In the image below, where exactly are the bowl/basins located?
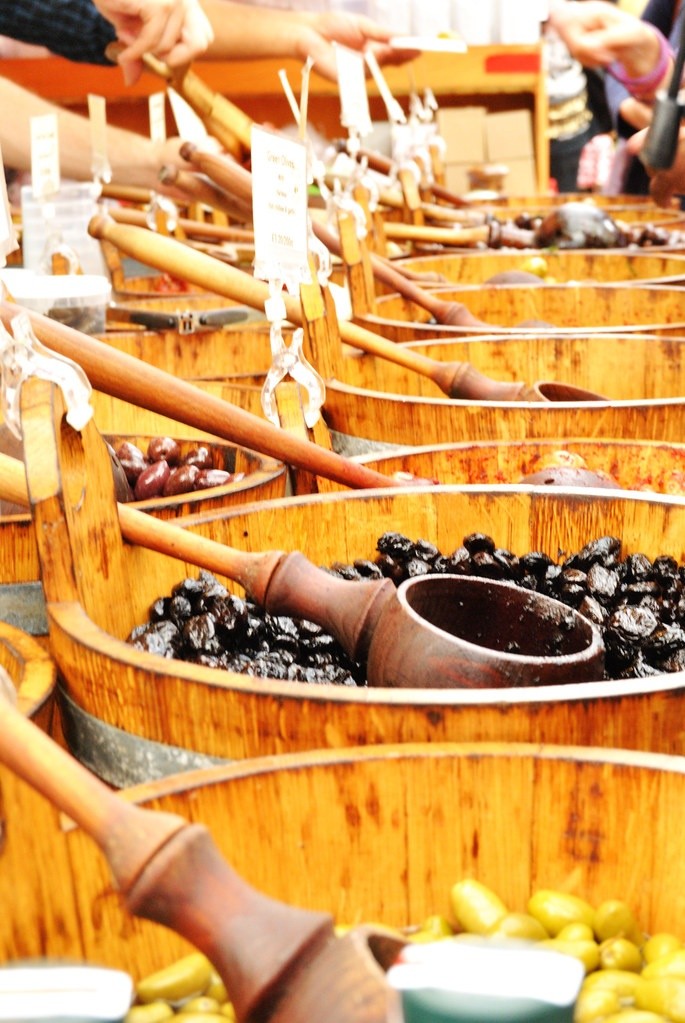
[3,178,683,1023]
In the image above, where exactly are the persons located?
[0,1,685,270]
